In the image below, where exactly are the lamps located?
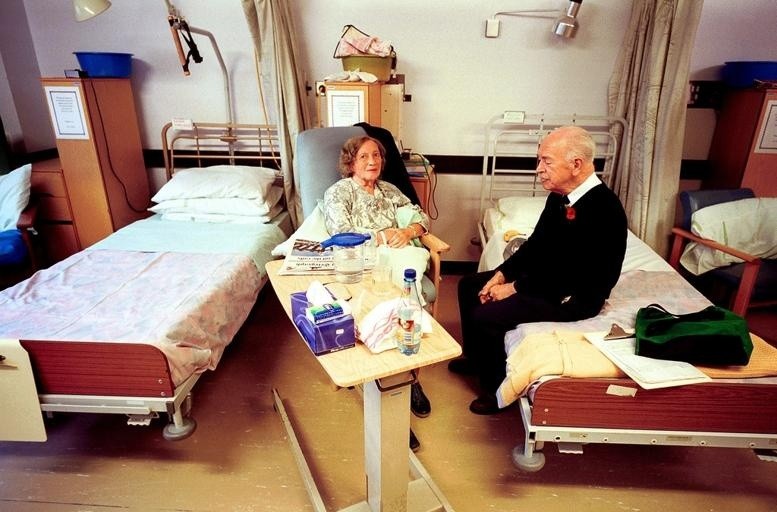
[482,1,580,47]
[70,0,202,74]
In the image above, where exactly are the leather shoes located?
[409,426,421,454]
[469,393,503,414]
[447,358,472,374]
[410,380,431,419]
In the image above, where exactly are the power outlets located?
[314,81,326,96]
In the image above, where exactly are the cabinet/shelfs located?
[318,78,381,130]
[399,162,437,214]
[26,156,79,264]
[38,75,153,271]
[706,86,776,197]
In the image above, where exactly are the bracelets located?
[408,225,417,238]
[379,231,387,245]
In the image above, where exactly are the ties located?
[559,194,570,209]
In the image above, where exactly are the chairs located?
[290,123,449,325]
[667,184,775,322]
[0,115,40,289]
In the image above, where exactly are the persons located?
[448,126,627,415]
[324,136,430,453]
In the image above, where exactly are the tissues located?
[290,280,355,356]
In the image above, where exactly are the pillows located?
[159,205,284,226]
[677,195,775,277]
[0,162,33,234]
[493,196,548,228]
[149,162,279,204]
[145,186,286,217]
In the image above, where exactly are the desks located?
[265,253,466,512]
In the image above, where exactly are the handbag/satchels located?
[633,298,754,369]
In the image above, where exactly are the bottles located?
[396,269,423,356]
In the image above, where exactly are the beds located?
[0,119,296,447]
[476,110,775,475]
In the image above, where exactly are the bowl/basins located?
[71,51,135,79]
